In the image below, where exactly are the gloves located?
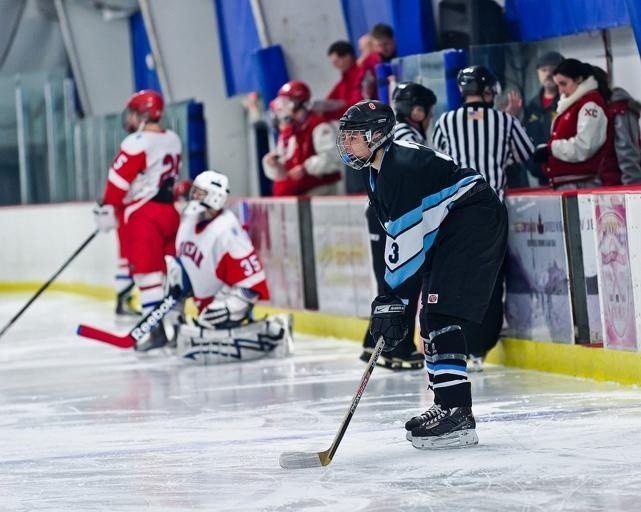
[369,296,409,353]
[195,298,231,329]
[93,201,119,232]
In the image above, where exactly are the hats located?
[535,51,566,69]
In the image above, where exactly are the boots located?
[116,294,142,317]
[132,324,178,350]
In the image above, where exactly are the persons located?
[335,100,508,449]
[609,87,641,186]
[524,52,563,139]
[165,170,294,363]
[357,24,397,71]
[535,59,623,189]
[262,82,345,196]
[320,41,375,195]
[432,65,550,373]
[92,88,188,356]
[359,82,439,369]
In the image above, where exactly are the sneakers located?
[405,404,448,432]
[268,313,292,355]
[364,347,425,363]
[411,405,477,436]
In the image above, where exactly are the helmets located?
[391,82,437,106]
[335,99,395,169]
[278,80,309,102]
[126,89,164,122]
[455,63,497,92]
[190,169,230,211]
